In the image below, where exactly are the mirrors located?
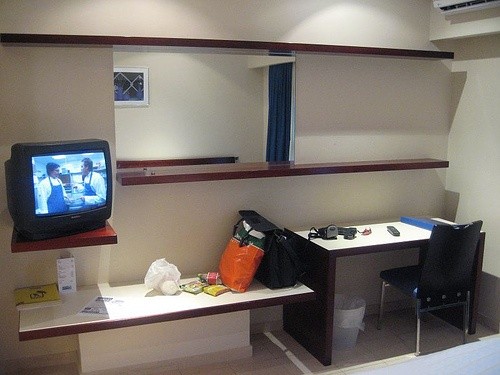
[112,44,453,177]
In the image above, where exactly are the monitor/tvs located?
[5,138,112,242]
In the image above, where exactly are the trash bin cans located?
[332,293,367,351]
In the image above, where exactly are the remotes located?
[387,226,400,236]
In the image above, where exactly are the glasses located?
[54,170,59,172]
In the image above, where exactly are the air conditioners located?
[433,0,500,16]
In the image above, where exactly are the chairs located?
[376,220,484,357]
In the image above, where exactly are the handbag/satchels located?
[238,210,306,290]
[218,218,265,293]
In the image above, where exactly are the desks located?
[282,218,486,367]
[18,277,317,375]
